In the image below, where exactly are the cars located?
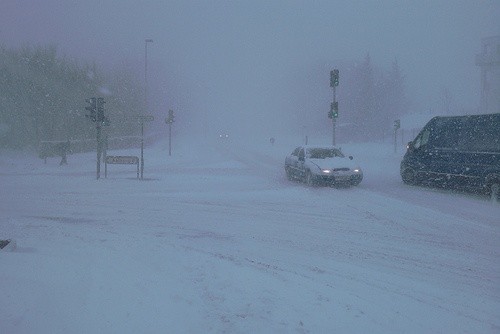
[285,146,363,188]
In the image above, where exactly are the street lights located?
[143,39,155,119]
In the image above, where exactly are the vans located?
[401,111,500,199]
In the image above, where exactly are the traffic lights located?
[394,119,400,129]
[329,69,339,87]
[85,97,106,123]
[327,102,339,119]
[169,110,176,124]
